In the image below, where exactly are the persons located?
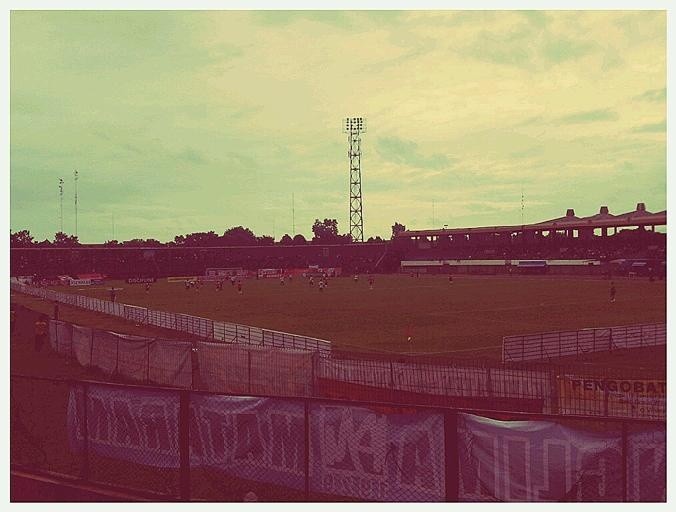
[10,307,16,343]
[33,317,47,350]
[12,244,667,303]
